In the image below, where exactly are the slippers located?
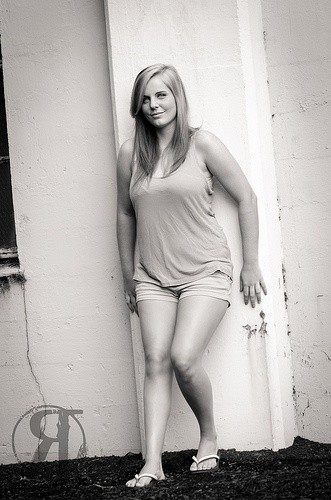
[125,474,166,488]
[189,455,220,474]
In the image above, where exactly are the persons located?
[116,63,268,490]
